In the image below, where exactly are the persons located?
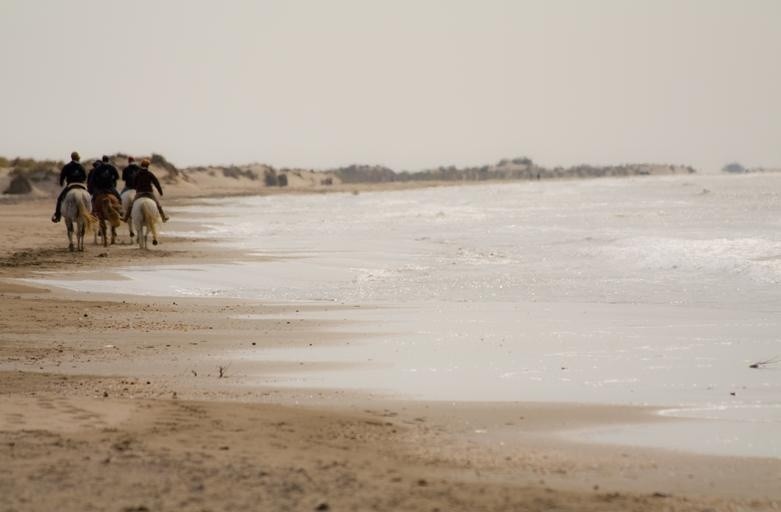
[87,159,104,195]
[119,158,170,224]
[52,151,87,223]
[91,154,123,205]
[118,155,140,195]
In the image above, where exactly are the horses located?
[131,197,159,251]
[92,195,120,248]
[60,188,93,252]
[120,189,137,244]
